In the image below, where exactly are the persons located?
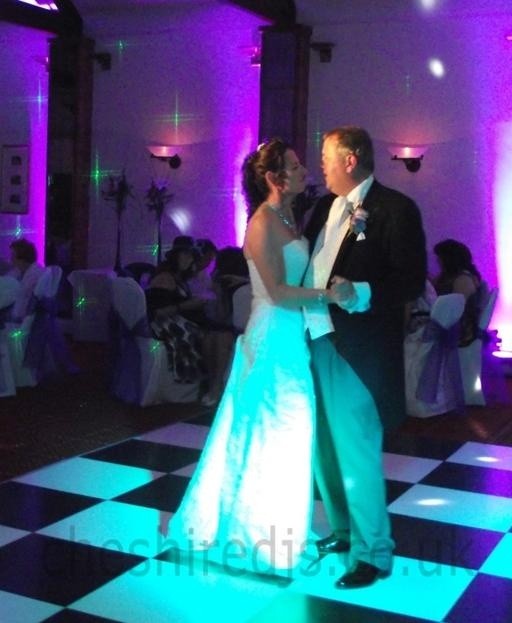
[0,239,49,322]
[434,238,488,353]
[168,138,358,577]
[300,128,427,588]
[141,237,237,406]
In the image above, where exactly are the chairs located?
[1,258,254,410]
[399,286,505,417]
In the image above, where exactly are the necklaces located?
[264,201,298,237]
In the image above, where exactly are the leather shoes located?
[316,535,349,551]
[336,558,390,589]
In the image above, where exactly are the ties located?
[324,196,347,245]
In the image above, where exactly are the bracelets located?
[317,288,325,306]
